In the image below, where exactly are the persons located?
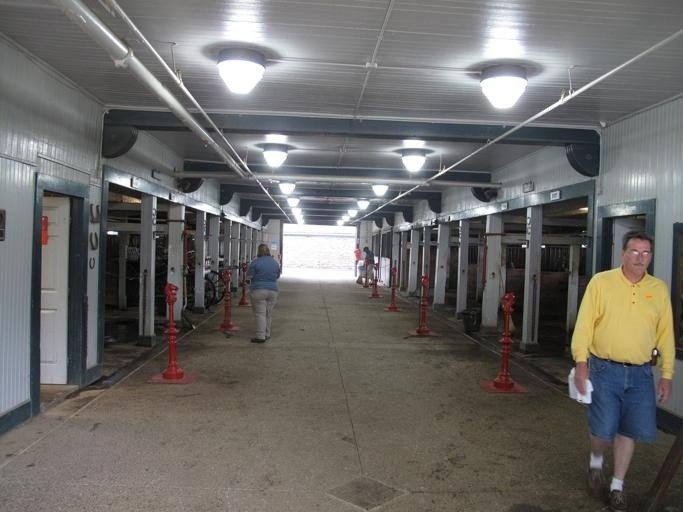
[353,247,361,277]
[570,230,676,512]
[363,247,375,285]
[246,244,281,343]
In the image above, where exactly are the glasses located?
[628,250,650,258]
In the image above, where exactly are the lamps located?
[336,209,359,225]
[286,196,300,208]
[481,66,527,110]
[372,184,389,197]
[216,49,264,94]
[401,149,426,173]
[357,198,370,211]
[279,180,295,195]
[264,144,289,168]
[291,208,305,224]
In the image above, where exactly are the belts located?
[611,361,632,368]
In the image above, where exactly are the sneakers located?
[250,336,270,343]
[586,456,604,498]
[607,488,628,512]
